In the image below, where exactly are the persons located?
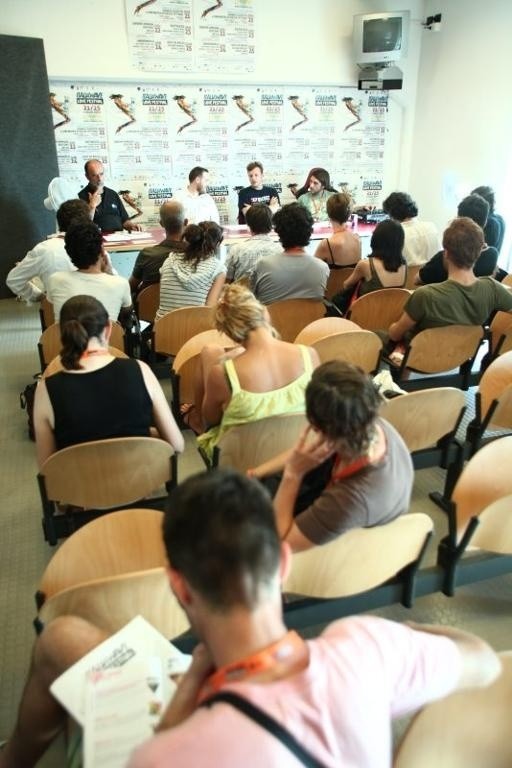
[32,295,186,520]
[245,359,416,554]
[7,159,512,365]
[1,469,504,768]
[178,282,321,470]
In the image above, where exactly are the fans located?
[43,176,77,234]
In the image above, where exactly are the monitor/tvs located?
[353,9,411,66]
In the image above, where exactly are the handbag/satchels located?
[20,382,37,442]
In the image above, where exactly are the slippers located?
[178,402,200,436]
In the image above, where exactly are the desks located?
[102,219,382,276]
